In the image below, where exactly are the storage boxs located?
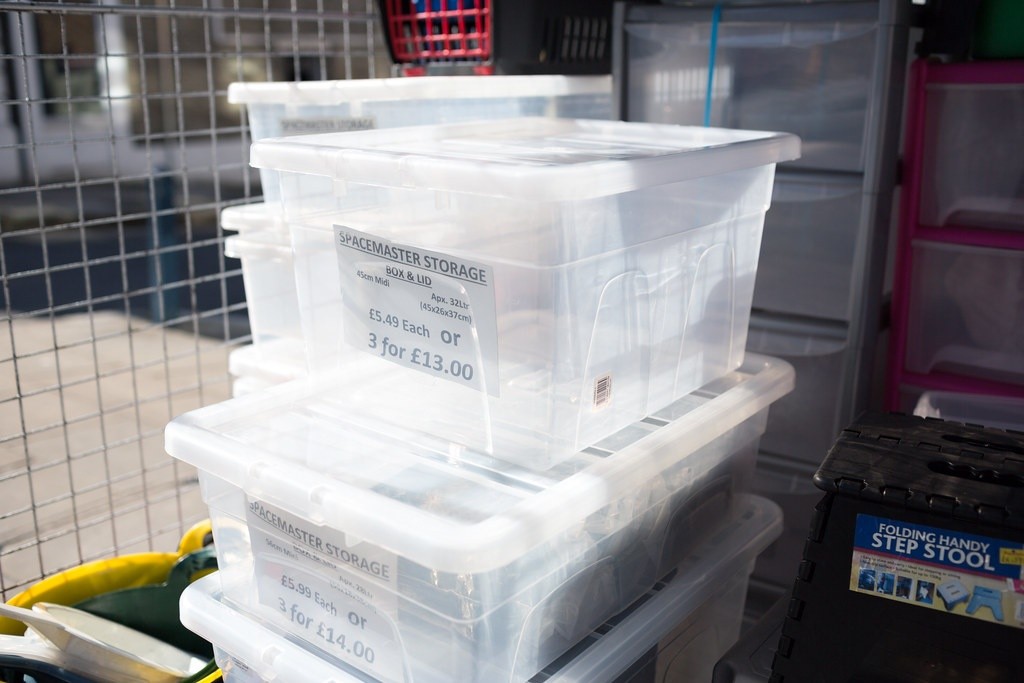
[165,0,1024,683]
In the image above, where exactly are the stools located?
[936,580,969,611]
[766,411,1024,683]
[965,585,1003,621]
